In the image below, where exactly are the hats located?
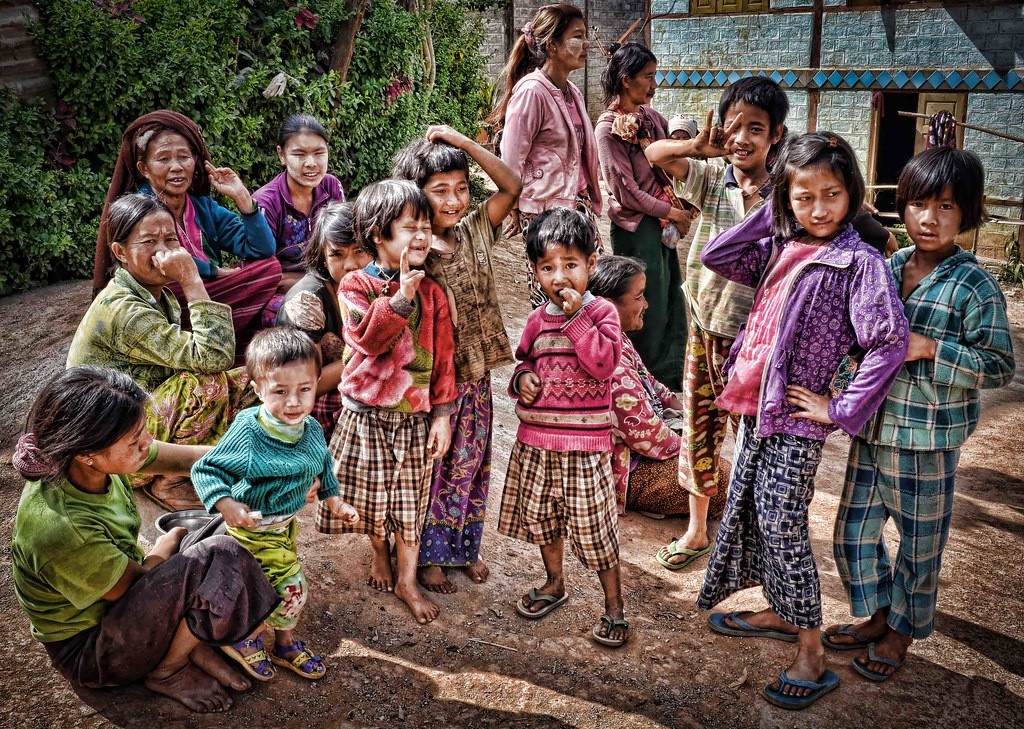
[666,114,697,139]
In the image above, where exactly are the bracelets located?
[337,502,346,519]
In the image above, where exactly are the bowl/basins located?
[155,508,221,540]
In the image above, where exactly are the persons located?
[696,130,910,709]
[821,145,1015,682]
[852,209,900,259]
[190,326,359,679]
[11,362,321,712]
[496,207,630,646]
[583,255,733,519]
[391,125,523,592]
[251,113,374,439]
[593,40,695,412]
[484,3,602,311]
[644,75,800,570]
[91,108,283,365]
[65,194,261,513]
[314,179,457,625]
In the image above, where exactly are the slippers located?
[593,614,629,646]
[516,587,569,618]
[761,668,840,709]
[851,643,907,682]
[655,541,711,570]
[707,611,799,643]
[821,623,871,649]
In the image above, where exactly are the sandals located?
[269,638,327,679]
[220,636,275,682]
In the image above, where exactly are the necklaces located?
[542,69,569,103]
[431,225,460,259]
[741,173,771,199]
[375,261,399,296]
